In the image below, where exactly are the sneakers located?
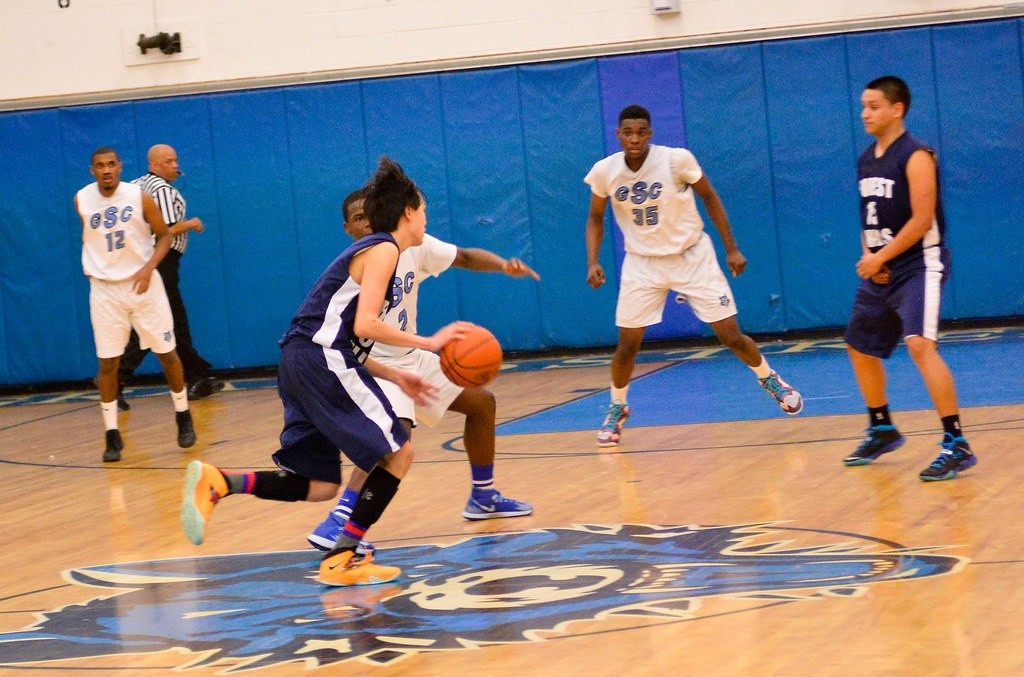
[318,550,402,586]
[842,424,906,467]
[180,460,229,546]
[596,401,631,447]
[102,429,125,463]
[307,510,376,556]
[175,410,197,448]
[919,432,978,481]
[461,491,533,519]
[757,369,804,416]
[95,376,130,411]
[187,377,225,401]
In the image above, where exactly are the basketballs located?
[437,323,504,388]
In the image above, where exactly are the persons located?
[92,144,225,409]
[583,106,803,447]
[306,189,540,558]
[843,75,978,481]
[74,147,196,462]
[183,154,474,586]
